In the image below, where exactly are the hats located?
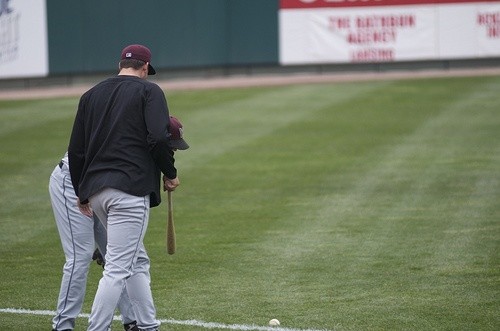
[121,45,156,75]
[169,115,190,150]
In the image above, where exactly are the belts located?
[59,161,64,168]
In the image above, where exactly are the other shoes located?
[124,320,140,331]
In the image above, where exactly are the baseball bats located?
[166,189,176,255]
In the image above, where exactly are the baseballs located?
[268,319,280,325]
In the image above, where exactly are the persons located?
[68,45,181,331]
[48,116,190,331]
